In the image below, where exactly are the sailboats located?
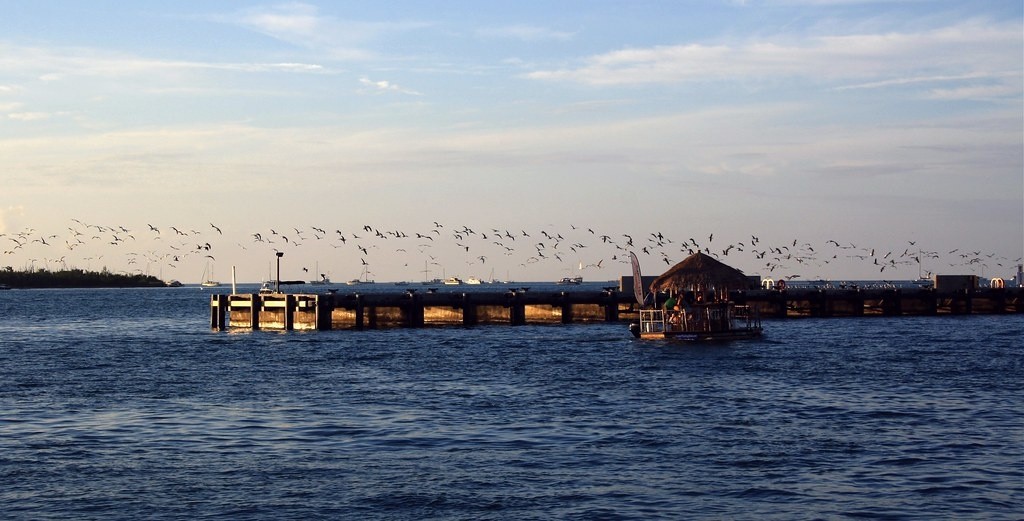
[310,261,330,285]
[483,267,515,285]
[421,260,432,285]
[199,260,220,287]
[358,263,375,284]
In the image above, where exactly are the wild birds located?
[0,219,1024,274]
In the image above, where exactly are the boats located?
[556,277,582,284]
[628,300,765,341]
[169,280,185,287]
[347,279,359,286]
[258,261,277,294]
[433,276,481,285]
[394,281,409,287]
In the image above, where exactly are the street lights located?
[276,252,284,293]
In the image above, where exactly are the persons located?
[664,293,703,325]
